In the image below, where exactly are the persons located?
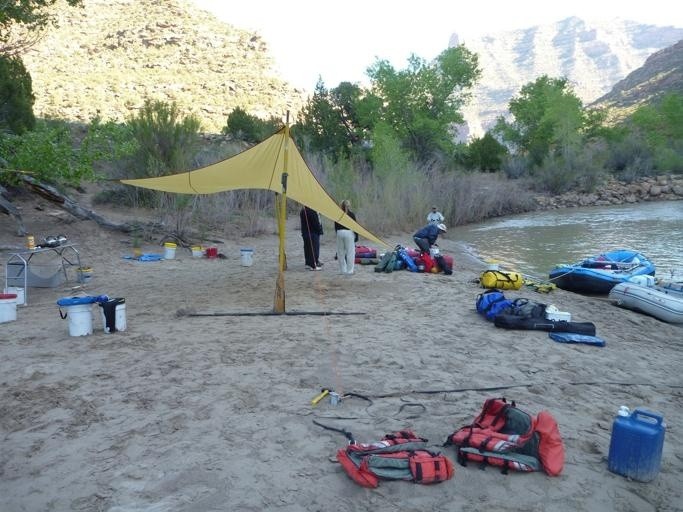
[332,198,357,276]
[426,205,445,246]
[298,205,324,271]
[411,222,447,255]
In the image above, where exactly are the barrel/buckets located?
[163,242,177,259]
[606,404,666,483]
[98,303,128,332]
[76,266,94,282]
[191,247,204,257]
[485,261,499,271]
[64,304,94,337]
[0,293,17,324]
[240,249,254,266]
[430,247,439,256]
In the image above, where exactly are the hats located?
[436,223,448,232]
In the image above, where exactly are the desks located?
[5,240,85,307]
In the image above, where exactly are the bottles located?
[25,232,34,249]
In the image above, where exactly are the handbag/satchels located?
[480,270,524,291]
[475,289,598,335]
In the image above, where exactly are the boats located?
[549,249,683,323]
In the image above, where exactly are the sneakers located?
[304,259,325,271]
